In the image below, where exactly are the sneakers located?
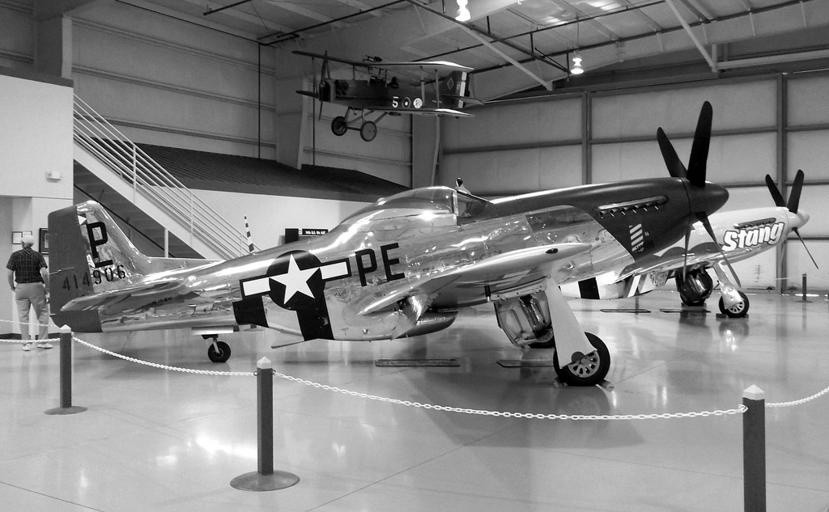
[37,342,54,349]
[21,343,31,352]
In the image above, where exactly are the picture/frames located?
[12,231,24,245]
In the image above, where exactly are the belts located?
[16,279,42,284]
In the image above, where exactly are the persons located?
[5,234,53,351]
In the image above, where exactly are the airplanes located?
[557,168,822,319]
[289,47,480,145]
[43,100,745,396]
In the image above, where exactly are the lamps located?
[454,0,471,22]
[569,51,585,75]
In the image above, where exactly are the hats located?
[20,235,35,244]
